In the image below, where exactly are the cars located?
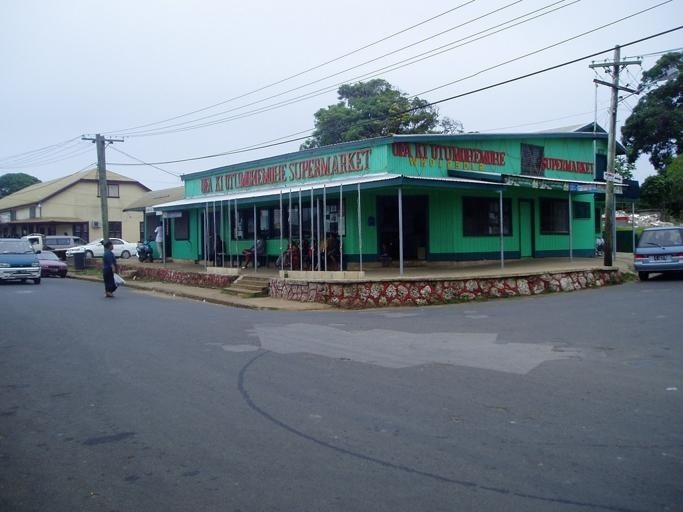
[35,251,68,278]
[65,238,137,260]
[633,226,682,281]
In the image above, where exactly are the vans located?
[45,235,88,249]
[0,238,41,284]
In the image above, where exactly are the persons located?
[233,211,245,239]
[279,231,342,271]
[240,237,265,269]
[152,221,163,259]
[100,241,118,298]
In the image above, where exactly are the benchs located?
[216,239,288,266]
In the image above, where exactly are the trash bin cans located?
[73,253,87,270]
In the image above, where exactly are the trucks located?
[21,233,81,260]
